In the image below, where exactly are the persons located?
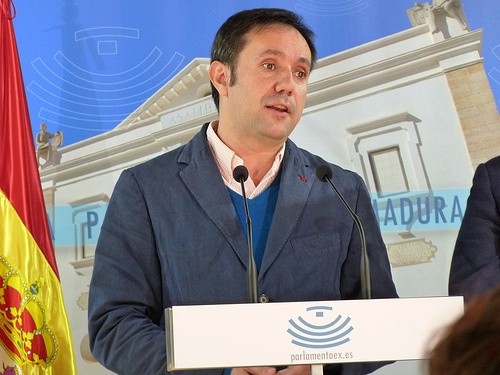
[448,154,500,302]
[87,8,400,375]
[423,285,500,375]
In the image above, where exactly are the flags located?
[0,0,75,375]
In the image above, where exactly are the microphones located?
[232,165,258,303]
[316,165,371,300]
